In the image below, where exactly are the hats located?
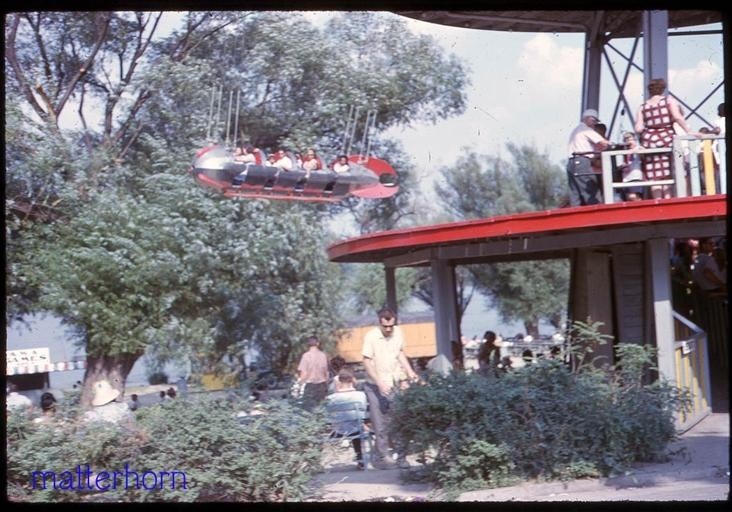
[582,108,601,123]
[91,380,120,406]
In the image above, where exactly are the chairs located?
[319,400,374,472]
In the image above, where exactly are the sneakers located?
[372,456,410,469]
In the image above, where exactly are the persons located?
[324,354,368,471]
[566,78,725,207]
[362,309,420,470]
[129,393,141,411]
[6,378,34,424]
[159,387,176,401]
[670,235,732,291]
[522,345,561,364]
[296,335,328,412]
[30,392,58,424]
[476,331,502,374]
[228,147,351,178]
